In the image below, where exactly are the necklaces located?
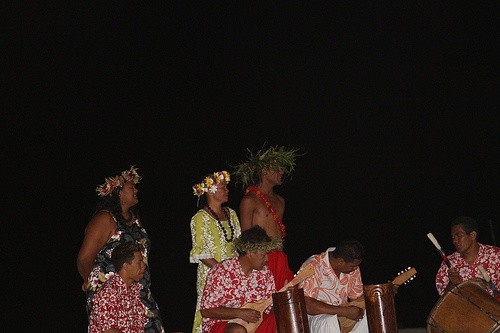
[206,204,236,243]
[123,208,136,226]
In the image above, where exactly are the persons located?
[189,170,243,333]
[87,243,149,333]
[296,239,399,333]
[76,166,168,333]
[434,216,500,333]
[239,149,297,333]
[200,225,295,333]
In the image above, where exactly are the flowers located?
[192,171,232,196]
[96,164,142,197]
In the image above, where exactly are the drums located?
[424,280,500,333]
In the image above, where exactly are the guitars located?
[338,266,417,333]
[228,263,316,333]
